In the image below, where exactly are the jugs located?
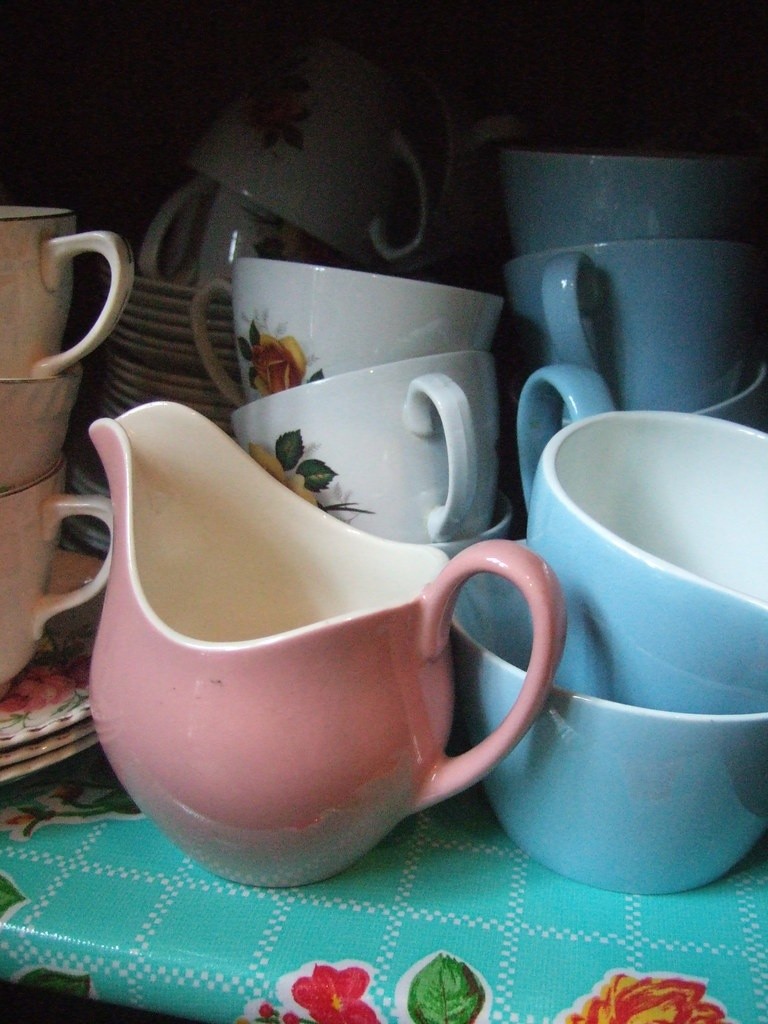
[90,401,565,890]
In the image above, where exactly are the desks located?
[1,746,766,1023]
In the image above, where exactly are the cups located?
[231,350,502,544]
[138,172,354,287]
[0,206,134,380]
[184,39,430,258]
[0,360,84,494]
[450,541,768,894]
[494,147,723,257]
[405,58,529,256]
[0,454,113,699]
[191,259,504,407]
[516,364,768,715]
[503,235,757,410]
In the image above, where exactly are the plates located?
[0,549,106,782]
[61,259,244,551]
